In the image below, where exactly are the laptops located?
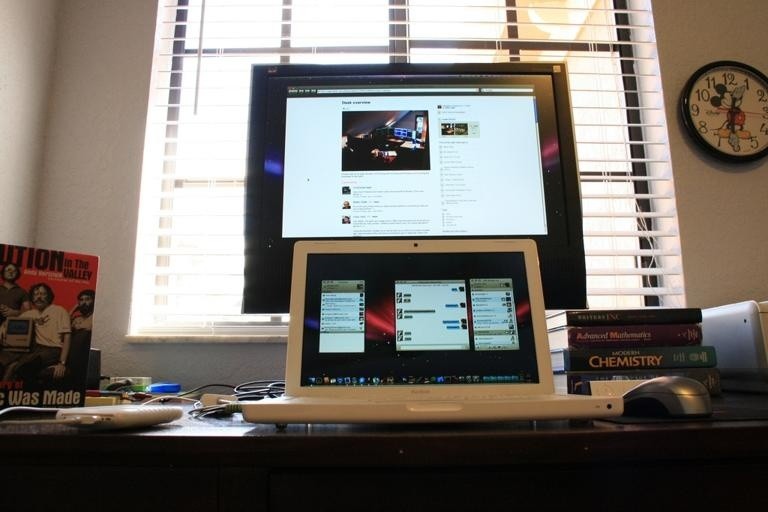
[238,239,625,423]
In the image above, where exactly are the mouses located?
[622,375,713,418]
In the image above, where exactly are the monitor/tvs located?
[240,60,588,311]
[394,128,408,140]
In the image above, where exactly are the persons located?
[1,261,31,335]
[68,290,96,359]
[2,280,73,384]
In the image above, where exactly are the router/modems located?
[55,404,184,429]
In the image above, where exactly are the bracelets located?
[59,360,67,366]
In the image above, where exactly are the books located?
[545,306,722,401]
[0,243,101,421]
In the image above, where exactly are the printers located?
[701,300,768,394]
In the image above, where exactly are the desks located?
[0,391,767,510]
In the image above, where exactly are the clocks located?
[676,58,768,163]
